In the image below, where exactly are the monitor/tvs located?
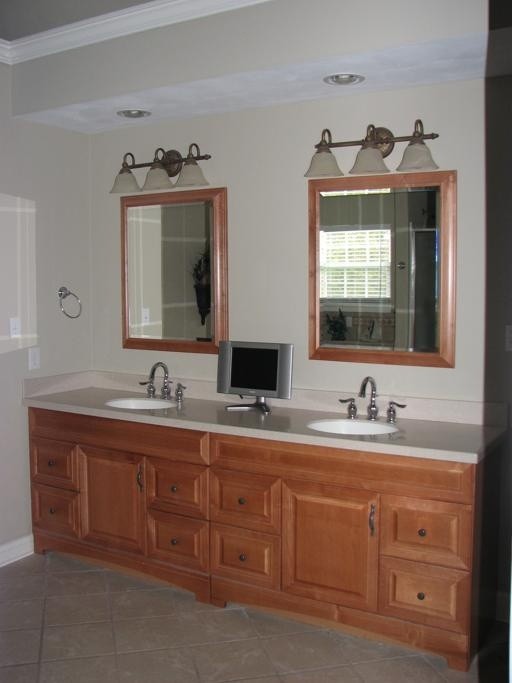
[217,340,294,414]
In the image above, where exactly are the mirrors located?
[304,167,459,371]
[116,184,231,355]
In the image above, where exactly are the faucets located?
[358,375,379,420]
[149,360,173,397]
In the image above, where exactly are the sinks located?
[106,398,181,412]
[307,416,398,436]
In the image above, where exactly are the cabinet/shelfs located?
[209,422,510,673]
[21,402,210,607]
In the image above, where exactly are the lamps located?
[304,114,442,184]
[108,140,214,200]
